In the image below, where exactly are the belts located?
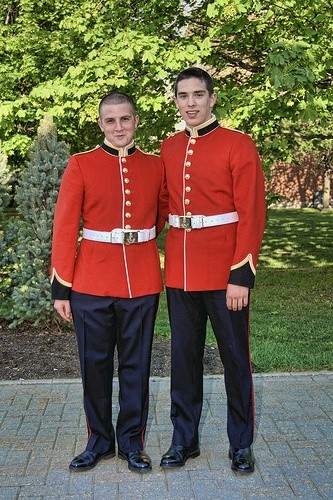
[82,225,156,244]
[169,210,239,229]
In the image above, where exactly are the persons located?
[155,66,269,474]
[48,90,171,476]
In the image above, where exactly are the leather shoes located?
[69,448,116,471]
[118,448,153,472]
[228,443,255,473]
[160,444,200,467]
